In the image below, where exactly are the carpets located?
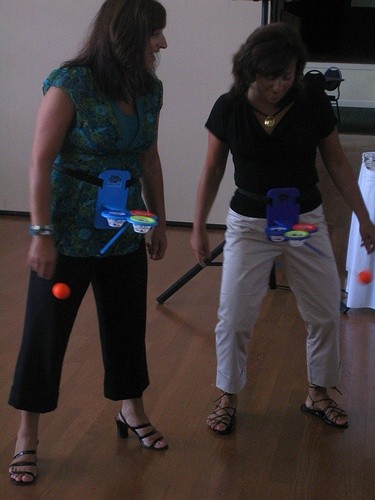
[332,106,375,135]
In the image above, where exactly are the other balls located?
[52,282,69,298]
[356,270,371,285]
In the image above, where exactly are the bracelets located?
[29,223,57,237]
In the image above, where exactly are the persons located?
[189,22,375,434]
[7,0,167,485]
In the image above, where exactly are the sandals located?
[301,387,349,428]
[208,390,237,434]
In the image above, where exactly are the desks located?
[345,151,375,309]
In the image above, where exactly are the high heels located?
[9,439,39,485]
[115,409,169,451]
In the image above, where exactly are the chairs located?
[305,65,344,124]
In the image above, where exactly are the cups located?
[131,210,155,218]
[267,226,286,242]
[292,223,317,232]
[130,215,156,233]
[285,230,308,247]
[107,211,126,227]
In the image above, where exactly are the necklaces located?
[254,101,289,127]
